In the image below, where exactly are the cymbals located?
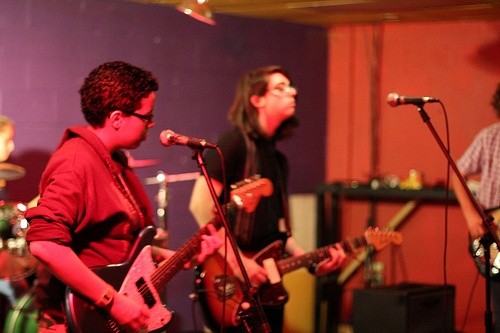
[0,162,26,182]
[125,155,162,168]
[136,172,202,188]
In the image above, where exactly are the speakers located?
[351,281,455,333]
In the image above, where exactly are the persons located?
[188,66,345,333]
[25,61,224,333]
[0,115,15,162]
[454,86,500,333]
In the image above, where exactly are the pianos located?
[313,179,475,207]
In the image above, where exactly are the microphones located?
[386,93,439,107]
[159,129,218,149]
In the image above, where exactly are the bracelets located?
[98,287,114,307]
[310,259,320,273]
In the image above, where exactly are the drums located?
[0,200,40,282]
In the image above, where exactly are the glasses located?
[131,111,154,124]
[266,86,295,96]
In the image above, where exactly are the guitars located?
[63,173,274,333]
[195,225,404,333]
